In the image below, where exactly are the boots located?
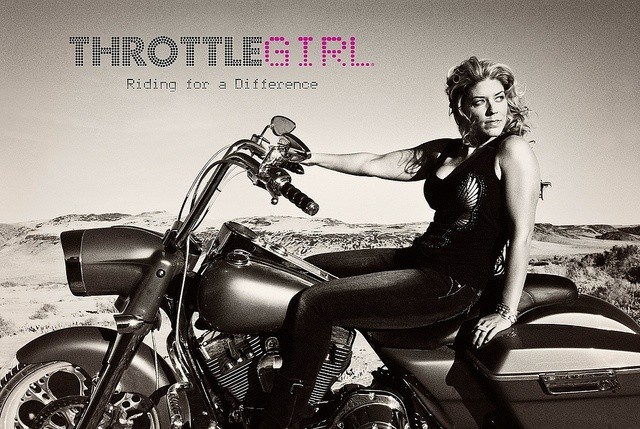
[251,371,323,429]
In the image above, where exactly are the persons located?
[256,56,541,429]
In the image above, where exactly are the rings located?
[478,326,489,333]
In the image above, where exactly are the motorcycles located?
[0,115,640,429]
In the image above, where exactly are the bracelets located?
[495,303,518,324]
